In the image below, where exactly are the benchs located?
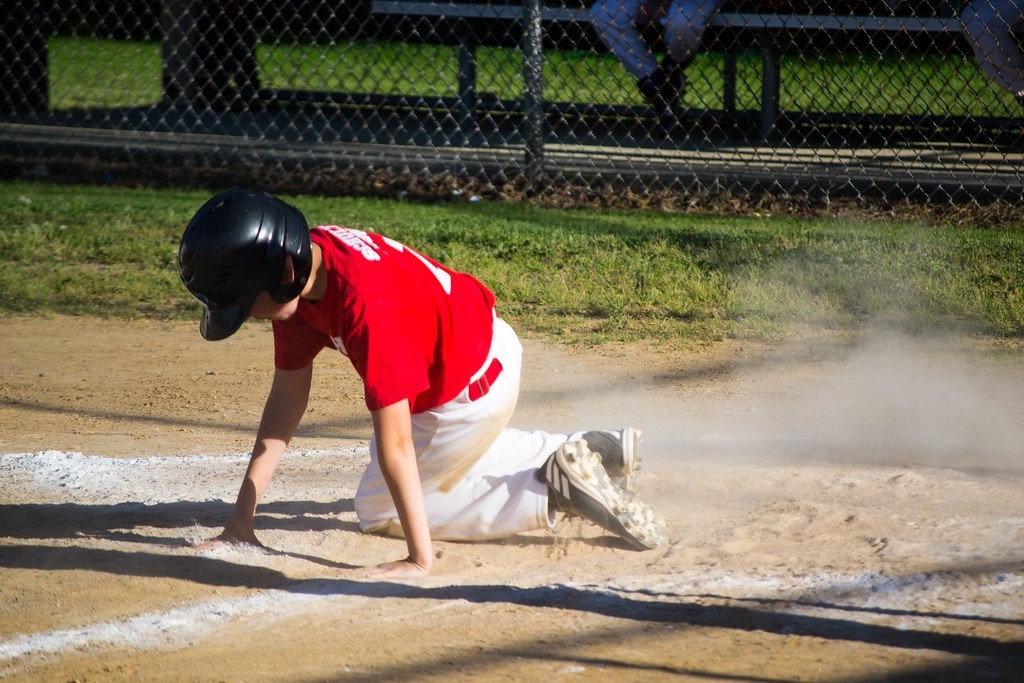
[371,0,1024,135]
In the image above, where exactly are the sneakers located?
[534,439,659,550]
[567,428,644,485]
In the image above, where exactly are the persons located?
[179,187,660,578]
[590,0,725,135]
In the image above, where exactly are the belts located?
[468,358,503,401]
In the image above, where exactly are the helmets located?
[178,185,309,341]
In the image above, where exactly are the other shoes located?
[636,68,679,139]
[660,52,697,84]
